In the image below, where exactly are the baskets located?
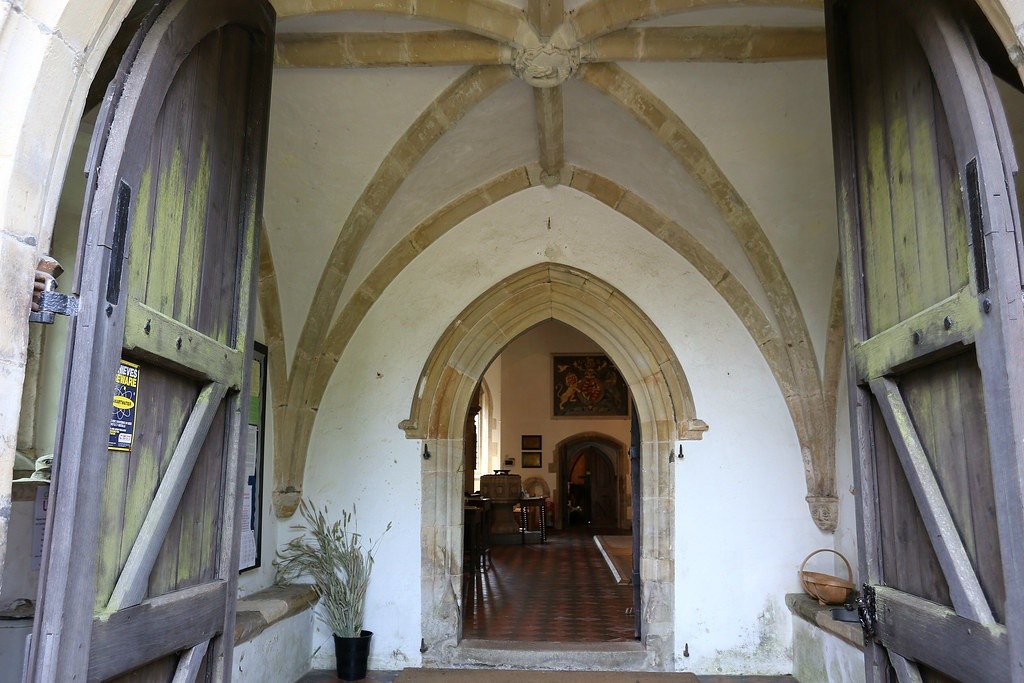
[798,548,856,604]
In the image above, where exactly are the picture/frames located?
[549,353,631,420]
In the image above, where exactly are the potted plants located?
[273,496,393,681]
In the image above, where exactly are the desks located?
[520,496,548,546]
[463,495,493,578]
